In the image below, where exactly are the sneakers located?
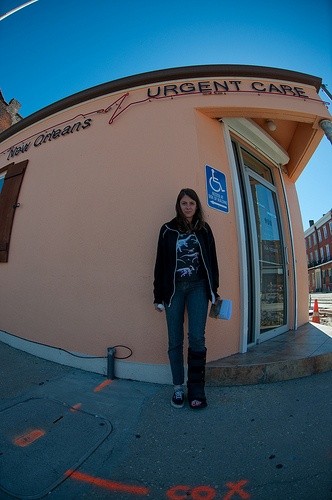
[171,390,185,408]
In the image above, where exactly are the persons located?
[153,189,221,409]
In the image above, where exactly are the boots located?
[188,347,207,409]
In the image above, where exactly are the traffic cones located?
[311,301,321,323]
